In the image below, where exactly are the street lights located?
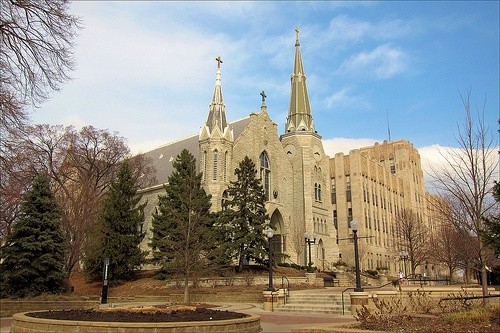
[400,251,408,279]
[304,232,318,273]
[266,228,278,292]
[349,220,365,292]
